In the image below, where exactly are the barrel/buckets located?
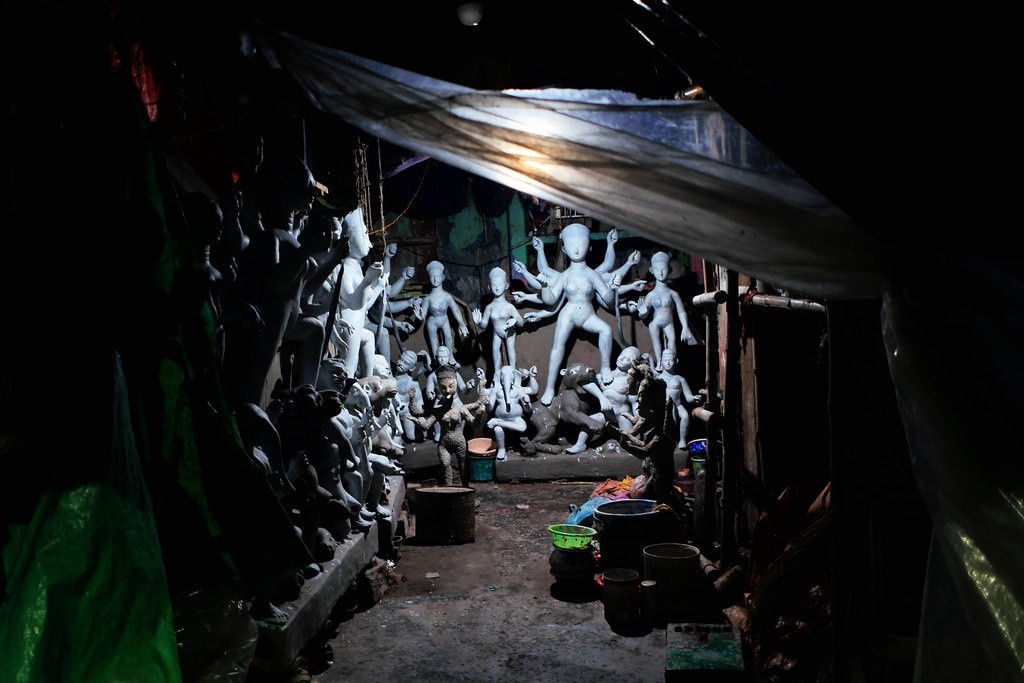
[601,569,637,620]
[594,499,665,568]
[414,486,476,546]
[692,456,706,481]
[468,455,496,481]
[642,543,700,606]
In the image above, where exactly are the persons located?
[180,124,703,628]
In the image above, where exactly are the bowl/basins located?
[547,525,597,550]
[467,438,496,456]
[687,439,707,457]
